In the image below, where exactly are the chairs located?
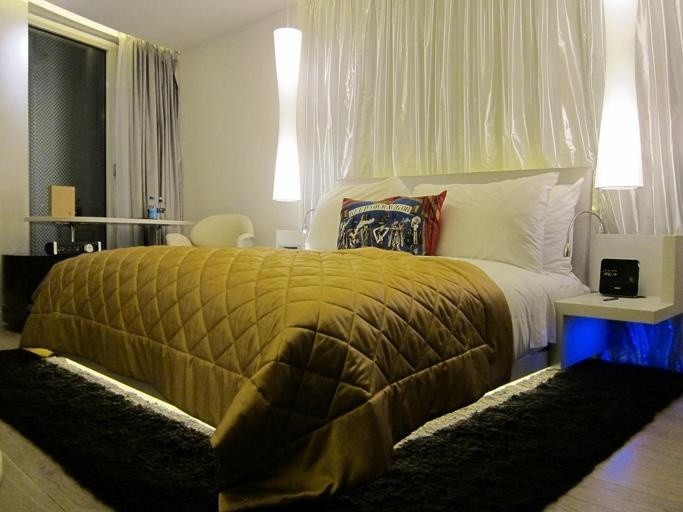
[163,214,255,247]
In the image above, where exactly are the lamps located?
[271,1,302,204]
[593,1,644,193]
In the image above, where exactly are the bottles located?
[146,195,166,220]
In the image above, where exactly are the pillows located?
[303,171,584,276]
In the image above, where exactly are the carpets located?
[0,346,682,511]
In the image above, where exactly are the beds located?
[21,165,595,459]
